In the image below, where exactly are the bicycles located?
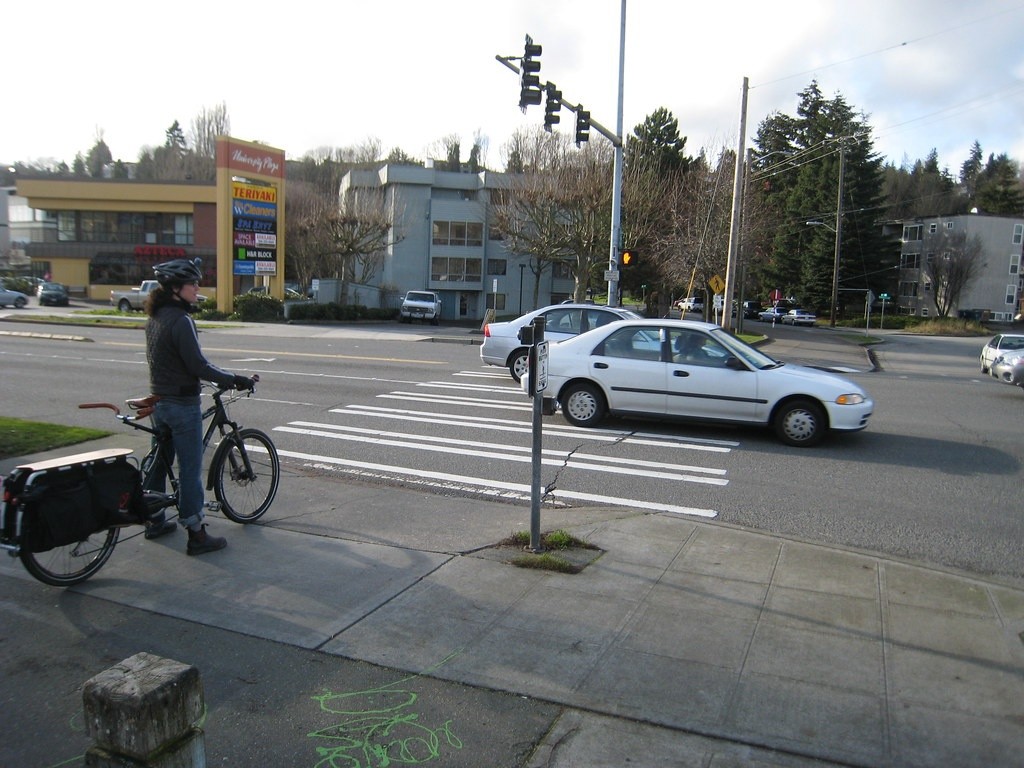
[0,373,281,588]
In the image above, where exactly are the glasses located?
[179,281,200,288]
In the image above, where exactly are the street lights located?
[806,221,842,328]
[735,148,794,334]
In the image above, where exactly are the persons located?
[688,332,735,363]
[140,260,227,555]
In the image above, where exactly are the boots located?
[187,523,228,555]
[144,512,178,539]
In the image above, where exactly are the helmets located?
[153,259,203,284]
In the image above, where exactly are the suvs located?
[398,289,443,327]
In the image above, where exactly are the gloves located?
[234,375,255,392]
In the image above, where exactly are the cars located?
[34,281,70,307]
[989,348,1024,390]
[244,286,313,303]
[478,302,730,385]
[0,284,31,310]
[520,316,875,449]
[676,295,818,327]
[979,333,1024,373]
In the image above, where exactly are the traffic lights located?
[574,105,592,150]
[544,81,564,135]
[518,34,546,113]
[618,248,638,268]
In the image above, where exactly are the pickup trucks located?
[109,279,208,312]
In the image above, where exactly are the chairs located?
[672,336,689,362]
[570,311,590,329]
